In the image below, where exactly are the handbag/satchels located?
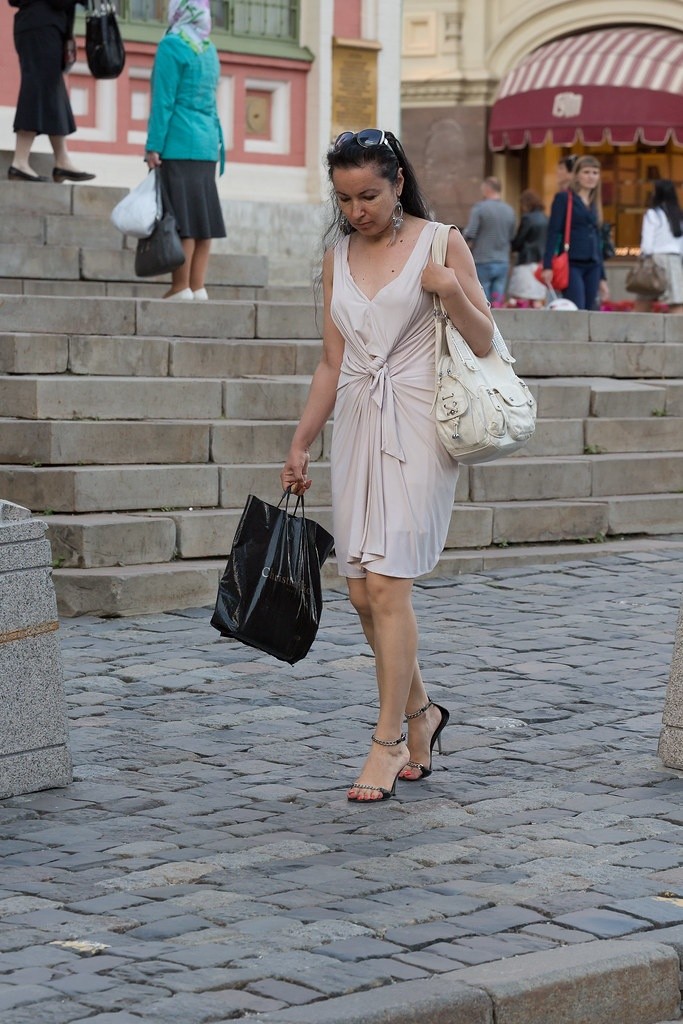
[84,0,126,80]
[429,222,537,466]
[65,38,77,72]
[111,166,186,277]
[626,258,668,296]
[534,249,569,288]
[211,485,334,666]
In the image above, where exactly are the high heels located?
[346,729,409,802]
[8,165,50,182]
[400,698,449,782]
[52,166,95,183]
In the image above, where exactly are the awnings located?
[488,26,683,148]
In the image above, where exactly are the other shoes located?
[161,286,208,302]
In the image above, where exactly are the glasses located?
[334,129,398,166]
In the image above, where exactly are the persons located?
[462,176,549,308]
[541,155,609,312]
[7,0,96,183]
[279,131,495,801]
[635,179,683,313]
[143,0,227,300]
